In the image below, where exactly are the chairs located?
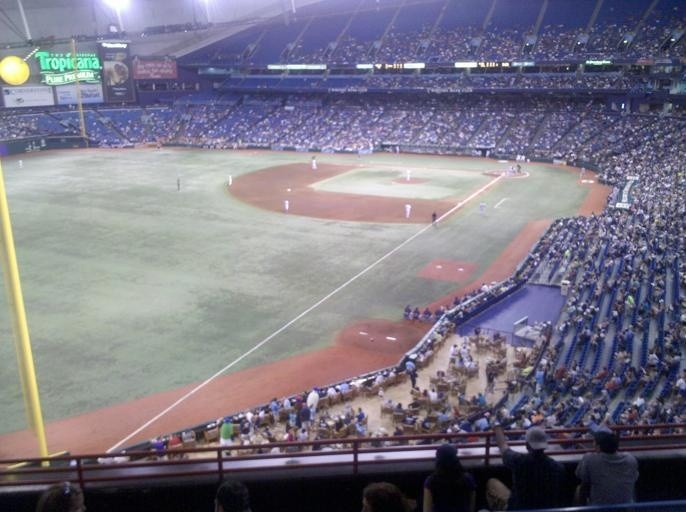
[96,179,686,465]
[197,0,686,48]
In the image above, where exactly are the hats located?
[588,423,619,451]
[435,444,459,462]
[525,426,548,451]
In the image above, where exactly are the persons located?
[424,443,478,511]
[487,415,571,508]
[403,157,686,451]
[284,199,289,212]
[17,159,22,168]
[311,156,317,170]
[69,363,402,465]
[36,480,86,512]
[361,481,416,511]
[177,177,180,192]
[572,420,640,507]
[213,479,251,512]
[228,174,232,186]
[1,1,686,158]
